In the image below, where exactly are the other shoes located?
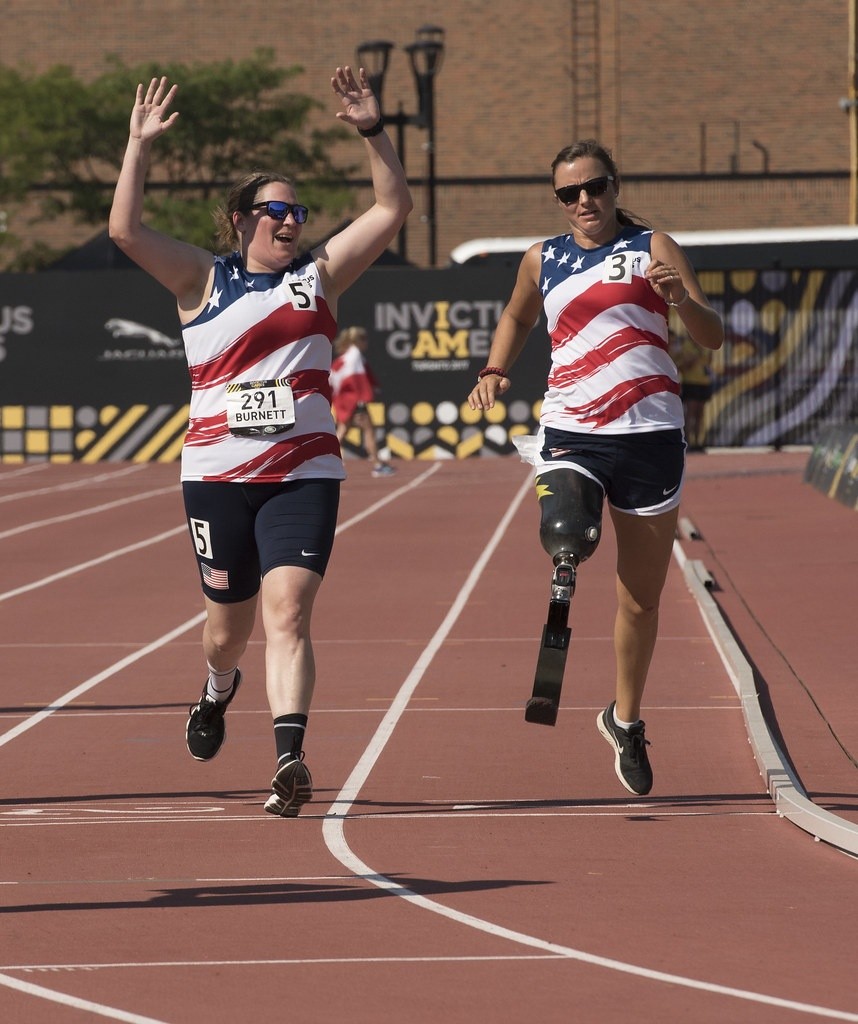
[371,463,398,478]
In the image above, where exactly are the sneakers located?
[595,700,654,796]
[264,746,313,818]
[186,667,242,761]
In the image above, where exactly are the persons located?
[109,64,414,817]
[668,330,714,455]
[329,326,399,477]
[468,140,725,795]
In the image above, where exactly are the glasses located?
[250,201,309,225]
[554,176,613,203]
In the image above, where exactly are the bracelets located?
[356,118,384,137]
[477,367,511,382]
[665,288,688,307]
